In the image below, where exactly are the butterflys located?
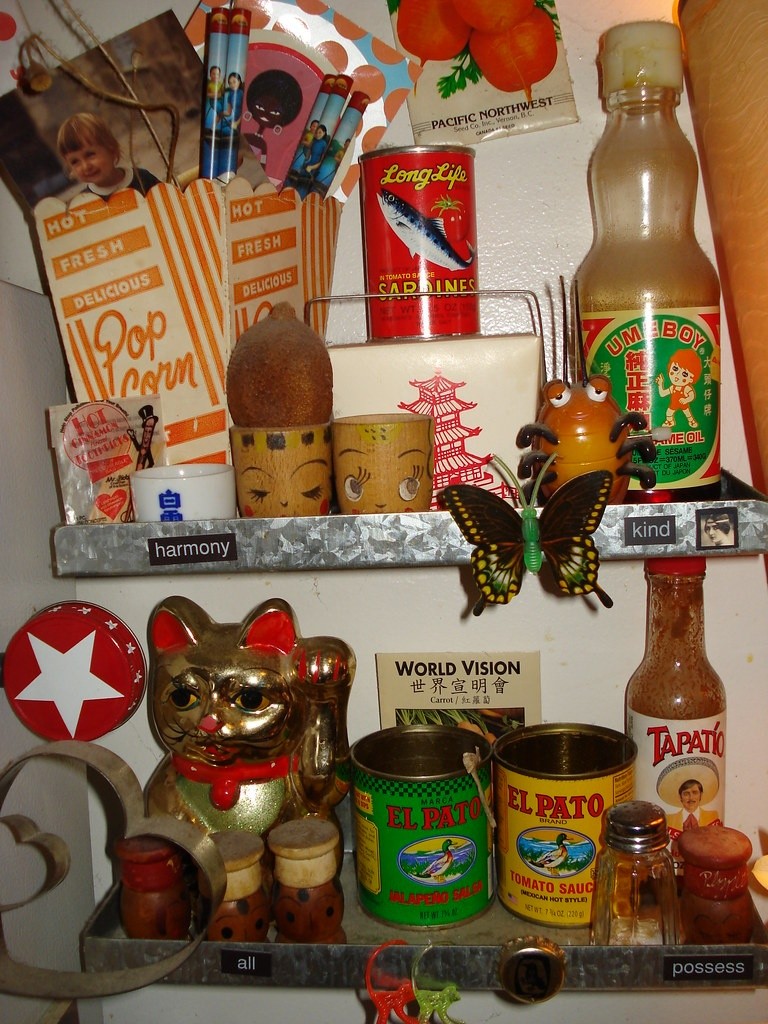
[440,457,613,621]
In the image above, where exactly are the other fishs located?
[376,188,477,272]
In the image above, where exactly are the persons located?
[205,65,224,131]
[301,120,320,156]
[56,112,160,203]
[334,139,351,171]
[298,125,328,176]
[221,70,244,135]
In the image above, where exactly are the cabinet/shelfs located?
[49,462,767,991]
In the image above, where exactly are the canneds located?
[359,146,483,341]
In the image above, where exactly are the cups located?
[332,413,434,514]
[229,420,332,518]
[129,463,237,521]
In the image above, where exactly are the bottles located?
[570,19,721,504]
[624,557,726,899]
[589,800,686,946]
